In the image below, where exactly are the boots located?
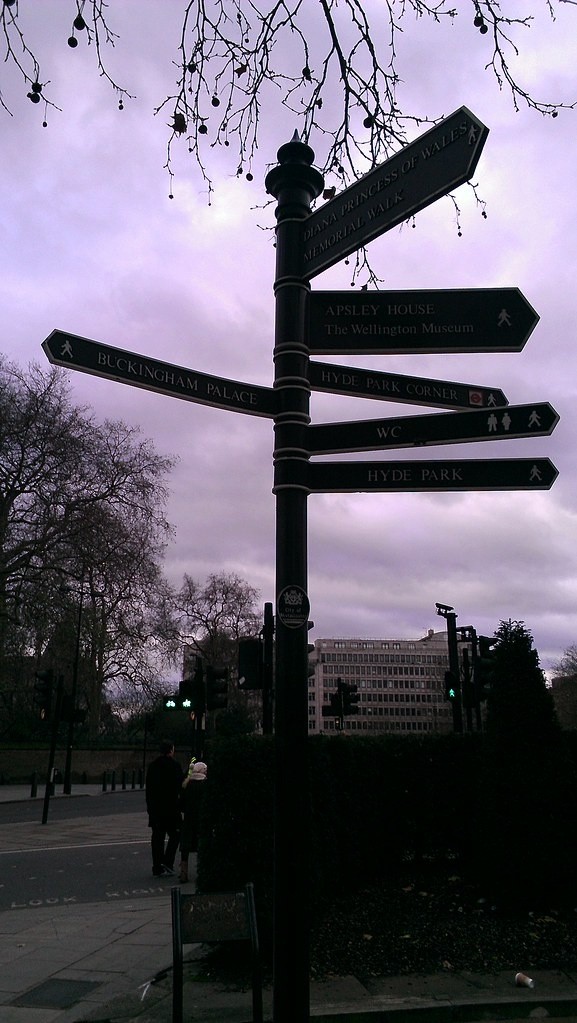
[179,861,188,882]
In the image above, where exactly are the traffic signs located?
[41,329,274,419]
[307,104,561,492]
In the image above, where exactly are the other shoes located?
[153,866,166,877]
[161,862,174,873]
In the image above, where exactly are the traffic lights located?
[206,664,228,710]
[479,636,497,658]
[335,718,340,729]
[147,718,155,731]
[475,656,493,697]
[163,680,196,711]
[33,669,52,721]
[307,621,315,677]
[445,669,459,700]
[343,684,359,716]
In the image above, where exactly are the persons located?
[146,740,186,878]
[177,757,208,883]
